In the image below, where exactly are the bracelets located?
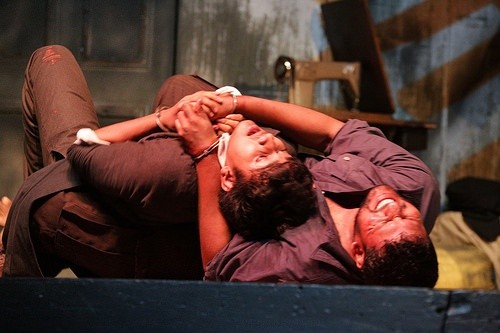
[231,95,237,114]
[193,140,219,160]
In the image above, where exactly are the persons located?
[0,44,439,288]
